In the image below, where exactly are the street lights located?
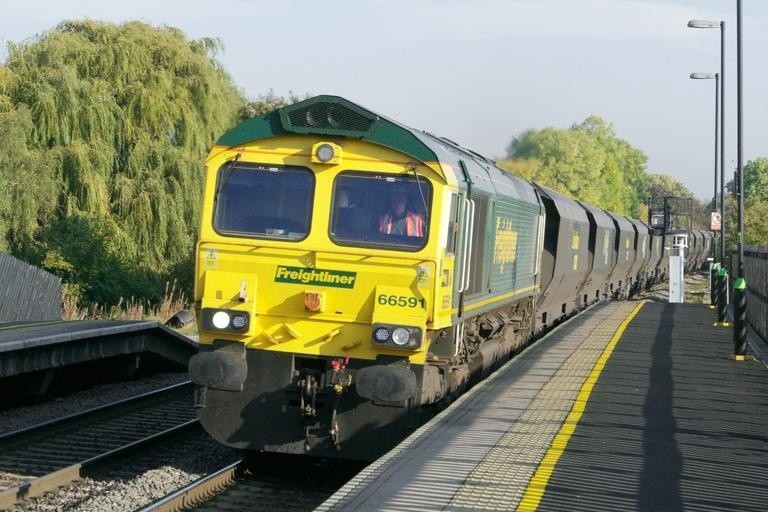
[687,18,730,326]
[690,71,721,309]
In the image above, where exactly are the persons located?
[369,190,425,238]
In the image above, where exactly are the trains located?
[182,95,724,461]
